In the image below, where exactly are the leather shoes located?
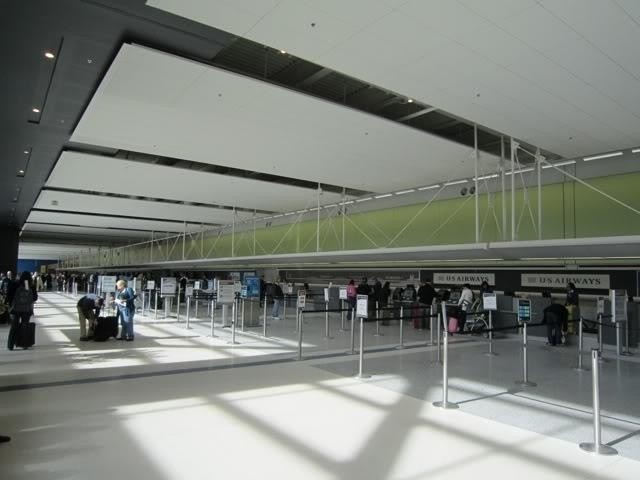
[79,336,92,341]
[119,337,134,341]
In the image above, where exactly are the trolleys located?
[462,297,489,338]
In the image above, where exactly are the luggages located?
[448,317,458,333]
[16,323,35,347]
[93,316,118,341]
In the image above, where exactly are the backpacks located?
[12,286,33,312]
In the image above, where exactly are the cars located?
[193,289,213,299]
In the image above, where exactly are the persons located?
[30,272,98,294]
[116,279,135,341]
[8,271,38,350]
[271,279,284,320]
[418,278,442,331]
[0,271,16,320]
[565,283,578,335]
[540,304,568,346]
[76,294,104,341]
[346,277,391,319]
[457,281,492,332]
